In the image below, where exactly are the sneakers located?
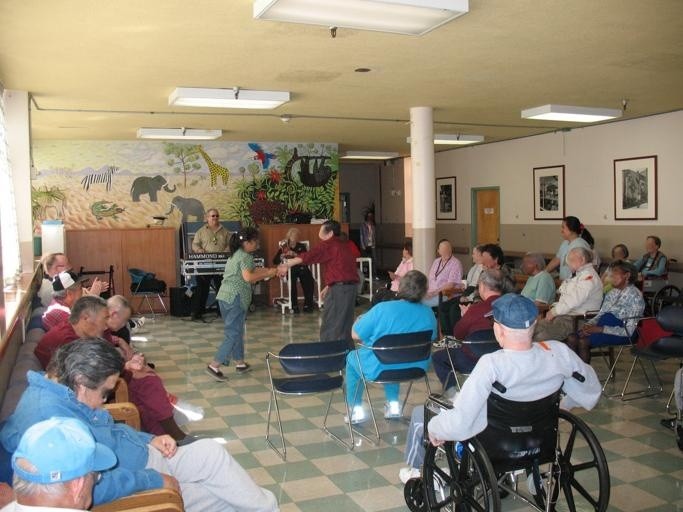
[205,365,228,382]
[344,406,366,424]
[383,401,402,419]
[433,338,456,348]
[235,363,250,374]
[294,307,299,313]
[303,307,313,312]
[399,468,421,484]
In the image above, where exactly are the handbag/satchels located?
[593,312,622,346]
[131,279,167,297]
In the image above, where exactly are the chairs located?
[1,440,184,510]
[441,329,503,398]
[349,331,433,446]
[438,274,683,416]
[1,284,142,430]
[266,340,354,461]
[129,268,168,320]
[272,240,322,315]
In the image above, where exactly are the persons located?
[1,251,196,445]
[372,243,412,305]
[344,270,437,423]
[204,228,277,382]
[273,228,316,314]
[0,417,117,511]
[399,293,602,490]
[277,220,360,341]
[426,239,516,347]
[360,212,378,271]
[521,216,668,363]
[431,270,507,397]
[0,337,277,512]
[660,369,683,430]
[191,209,230,321]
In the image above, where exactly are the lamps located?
[252,0,468,38]
[521,104,622,123]
[168,87,289,111]
[136,128,222,140]
[339,151,399,160]
[406,134,485,144]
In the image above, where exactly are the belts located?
[343,282,353,285]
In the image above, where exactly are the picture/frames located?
[435,176,457,220]
[614,156,658,219]
[533,166,565,220]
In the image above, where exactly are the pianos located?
[181,253,264,276]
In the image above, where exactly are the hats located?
[11,417,117,484]
[53,269,89,291]
[484,294,538,329]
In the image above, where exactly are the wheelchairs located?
[660,387,682,452]
[403,372,611,511]
[635,257,682,318]
